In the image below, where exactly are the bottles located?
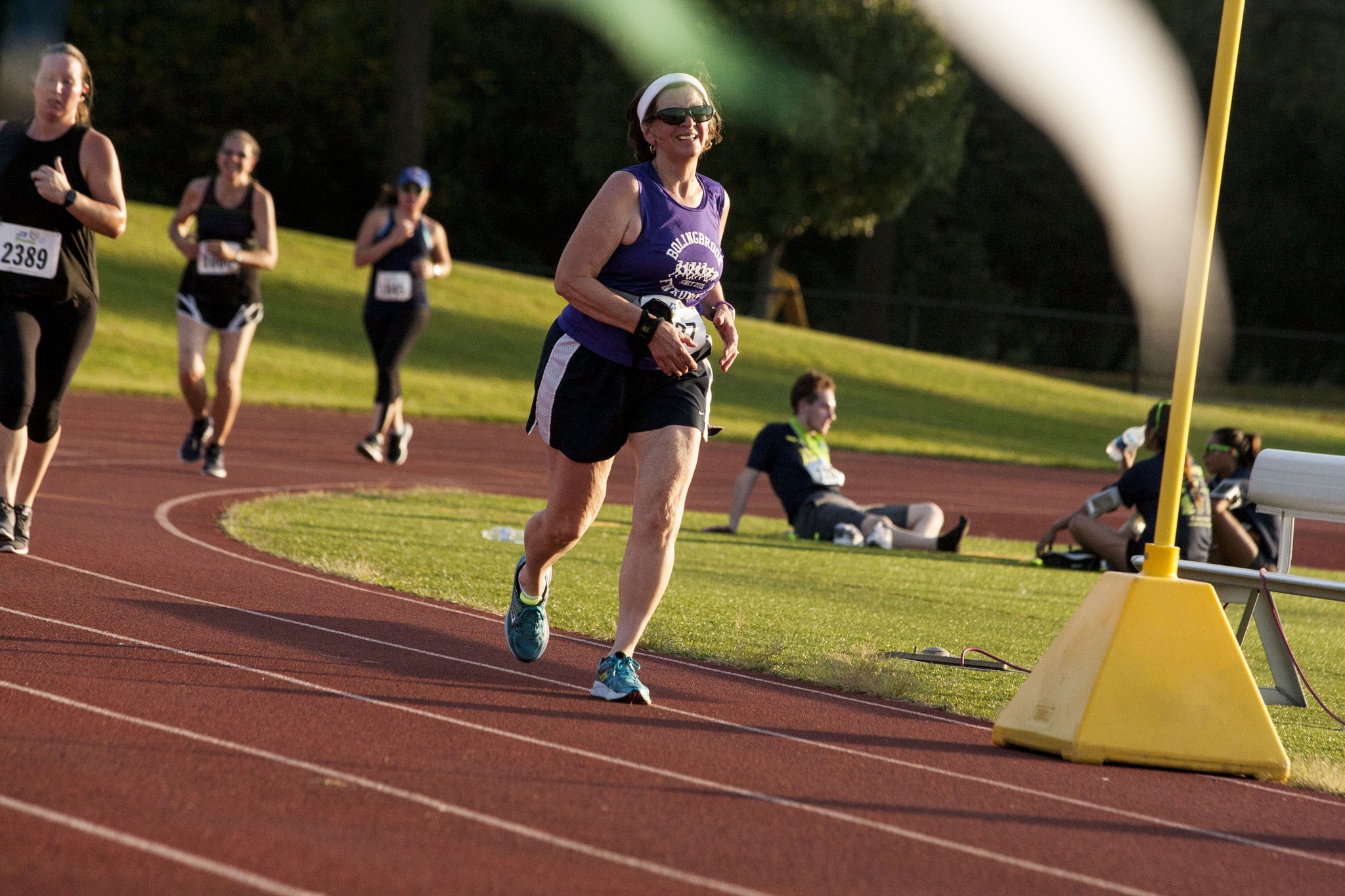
[481,526,525,546]
[1105,423,1148,462]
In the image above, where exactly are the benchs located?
[1130,448,1345,708]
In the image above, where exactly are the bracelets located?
[634,298,673,344]
[235,252,244,263]
[709,301,735,322]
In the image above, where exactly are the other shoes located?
[832,523,864,546]
[357,431,385,465]
[867,521,893,550]
[386,422,413,465]
[1042,549,1100,571]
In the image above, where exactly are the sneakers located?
[504,551,551,664]
[181,416,212,461]
[0,503,32,556]
[202,444,227,479]
[589,651,653,705]
[0,496,16,542]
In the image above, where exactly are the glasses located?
[648,104,714,126]
[1156,400,1170,429]
[1205,442,1237,457]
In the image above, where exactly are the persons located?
[1,43,128,555]
[702,371,971,554]
[1035,402,1213,574]
[504,71,741,707]
[350,167,454,468]
[1201,426,1283,573]
[166,131,280,479]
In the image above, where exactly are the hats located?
[399,168,432,191]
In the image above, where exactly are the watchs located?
[61,189,77,209]
[433,262,441,279]
